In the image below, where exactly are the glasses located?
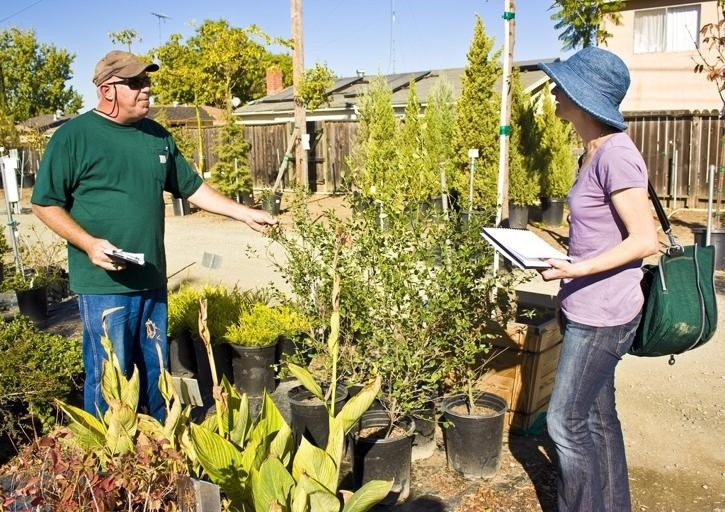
[104,75,151,90]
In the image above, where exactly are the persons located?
[536,46,661,512]
[28,49,279,428]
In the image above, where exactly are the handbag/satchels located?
[629,244,718,358]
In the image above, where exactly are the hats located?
[92,51,159,88]
[540,46,630,132]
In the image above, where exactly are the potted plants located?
[172,78,283,218]
[423,206,539,478]
[3,222,70,328]
[257,174,437,499]
[692,2,724,272]
[337,14,577,267]
[169,277,318,398]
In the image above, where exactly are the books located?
[479,226,573,272]
